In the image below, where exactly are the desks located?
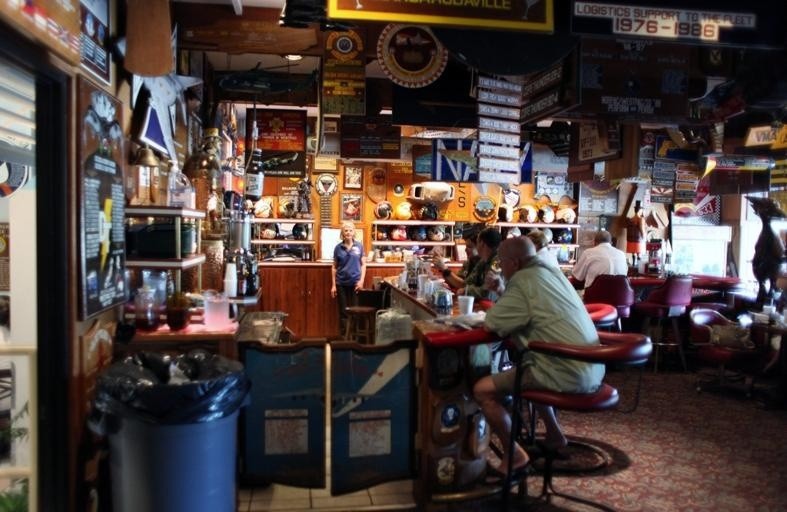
[129,301,242,364]
[751,322,787,361]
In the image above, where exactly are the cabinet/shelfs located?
[257,265,339,339]
[125,206,582,319]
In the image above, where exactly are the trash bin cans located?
[87,351,251,512]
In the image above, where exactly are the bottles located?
[133,284,160,334]
[238,259,261,298]
[244,147,266,202]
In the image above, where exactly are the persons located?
[329,221,367,335]
[627,200,646,268]
[433,226,516,456]
[571,230,629,299]
[474,237,605,478]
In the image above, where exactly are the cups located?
[201,289,239,332]
[457,295,476,316]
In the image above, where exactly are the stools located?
[345,306,378,346]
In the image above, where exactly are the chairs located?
[479,269,757,512]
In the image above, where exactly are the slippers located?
[527,441,571,460]
[484,457,533,482]
